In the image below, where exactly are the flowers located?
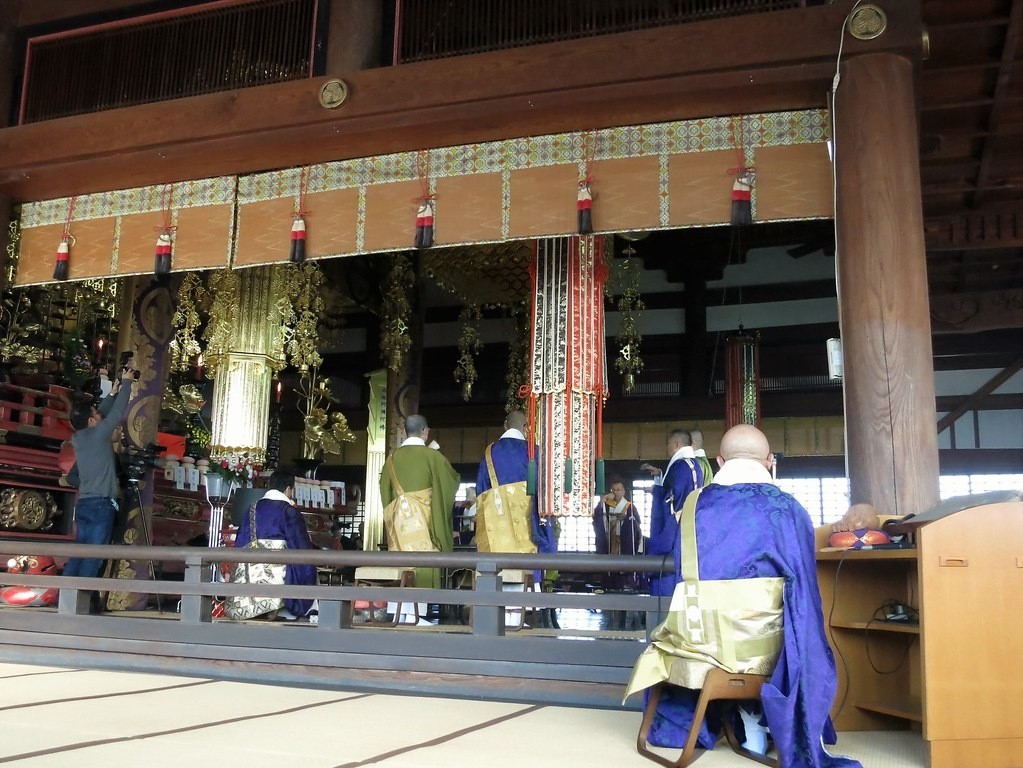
[205,445,261,486]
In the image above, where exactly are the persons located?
[622,424,862,768]
[475,409,560,614]
[61,368,134,578]
[639,427,714,596]
[223,470,317,620]
[592,481,641,591]
[380,415,462,589]
[331,522,364,581]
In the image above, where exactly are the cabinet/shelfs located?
[42,295,81,374]
[815,502,1023,768]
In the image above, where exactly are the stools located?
[349,566,419,627]
[469,569,535,631]
[637,659,781,768]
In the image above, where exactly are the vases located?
[204,473,230,497]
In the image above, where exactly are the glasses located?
[91,410,101,417]
[611,488,625,493]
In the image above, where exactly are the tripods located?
[100,468,163,616]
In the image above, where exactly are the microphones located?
[146,444,167,452]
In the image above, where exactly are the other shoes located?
[405,614,434,626]
[275,610,298,621]
[89,596,102,613]
[737,729,770,756]
[392,613,406,622]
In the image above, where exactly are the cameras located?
[117,351,140,383]
